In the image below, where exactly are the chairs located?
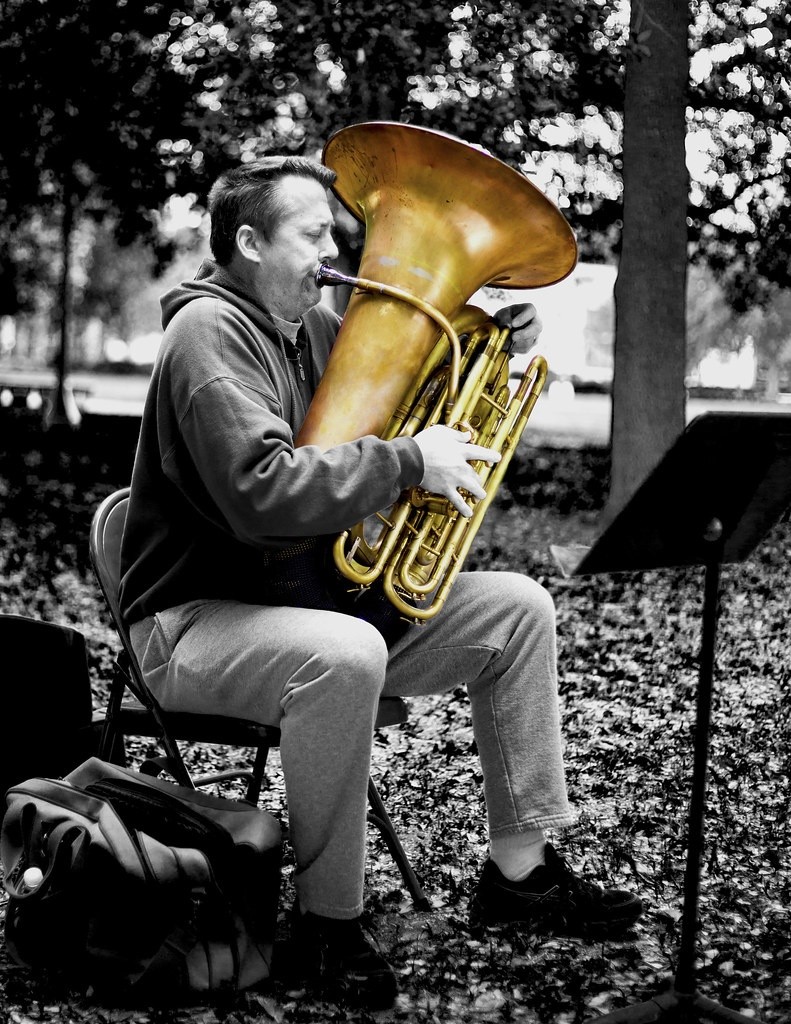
[88,488,433,923]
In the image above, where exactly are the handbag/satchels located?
[0,756,283,1003]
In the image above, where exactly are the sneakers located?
[471,841,643,932]
[288,897,399,1000]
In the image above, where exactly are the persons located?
[117,157,642,1011]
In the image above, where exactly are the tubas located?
[295,119,579,621]
